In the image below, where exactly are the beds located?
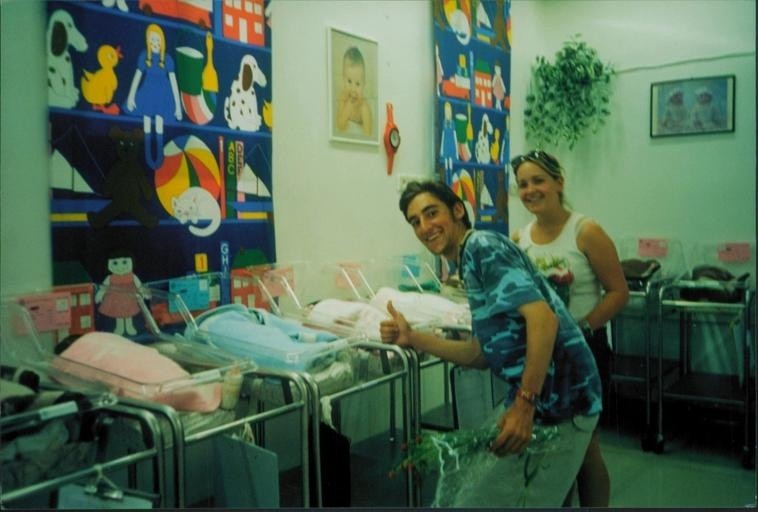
[10,284,307,508]
[606,239,691,455]
[1,365,166,511]
[248,260,471,510]
[136,273,415,510]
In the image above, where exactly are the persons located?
[378,179,604,507]
[508,149,628,507]
[662,86,720,130]
[334,46,372,141]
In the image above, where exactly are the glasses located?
[509,150,541,169]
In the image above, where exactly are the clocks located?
[383,100,402,177]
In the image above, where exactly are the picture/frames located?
[325,25,381,148]
[649,73,736,141]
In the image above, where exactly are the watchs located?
[516,386,539,407]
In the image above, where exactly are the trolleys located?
[592,260,683,454]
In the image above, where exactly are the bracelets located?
[578,319,594,339]
